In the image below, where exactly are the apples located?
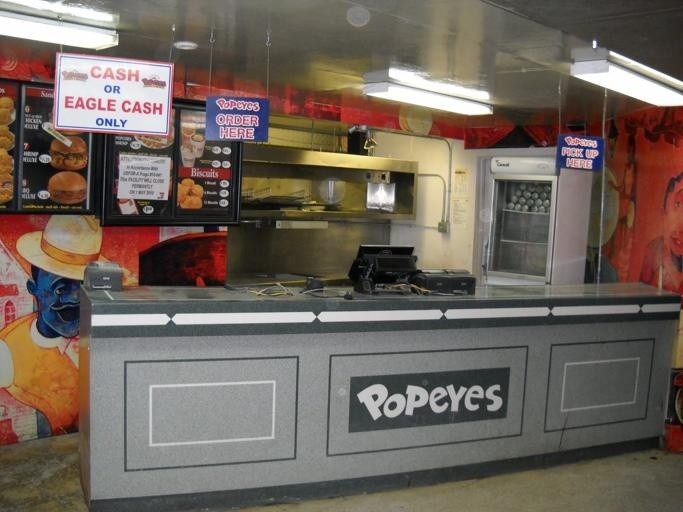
[507,181,551,213]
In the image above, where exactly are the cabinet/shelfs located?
[235,145,419,220]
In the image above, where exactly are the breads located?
[177,178,206,210]
[0,96,16,203]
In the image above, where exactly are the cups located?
[181,126,205,167]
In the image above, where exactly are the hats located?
[16,214,132,282]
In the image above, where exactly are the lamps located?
[566,57,683,109]
[362,81,494,120]
[0,11,120,54]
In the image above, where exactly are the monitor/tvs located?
[349,245,414,286]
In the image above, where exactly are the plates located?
[133,124,174,150]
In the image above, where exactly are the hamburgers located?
[48,135,87,170]
[46,172,88,205]
